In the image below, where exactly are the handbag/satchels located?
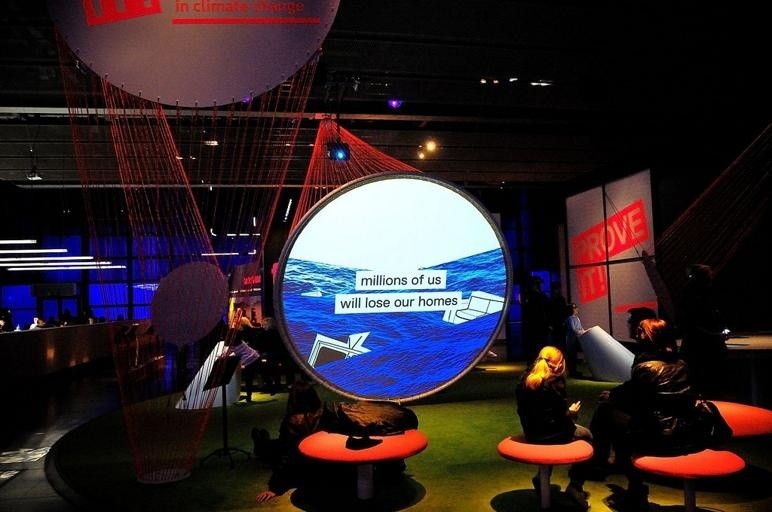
[691,398,733,448]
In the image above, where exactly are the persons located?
[520,276,583,377]
[1,310,104,330]
[583,319,694,510]
[235,317,293,400]
[517,346,589,501]
[627,308,655,339]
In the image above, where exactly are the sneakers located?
[565,485,590,510]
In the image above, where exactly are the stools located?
[497,398,772,511]
[298,426,429,500]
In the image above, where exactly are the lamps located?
[329,143,352,163]
